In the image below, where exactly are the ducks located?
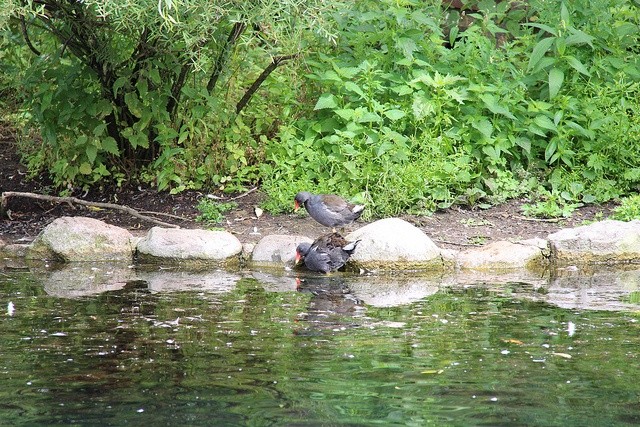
[294,232,364,277]
[294,190,369,235]
